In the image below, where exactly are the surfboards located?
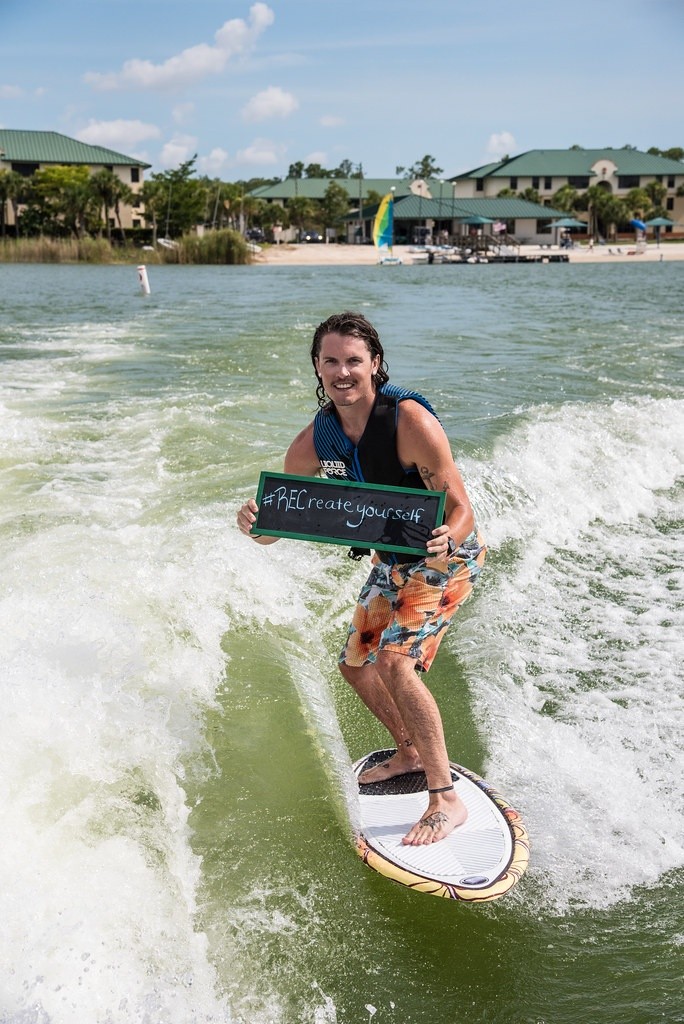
[346,745,530,903]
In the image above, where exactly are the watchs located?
[448,536,456,554]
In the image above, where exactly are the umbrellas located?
[631,220,645,230]
[645,218,676,247]
[546,219,588,250]
[459,215,495,224]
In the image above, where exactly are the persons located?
[238,312,486,846]
[637,230,648,254]
[587,234,595,253]
[441,228,449,244]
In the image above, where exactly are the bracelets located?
[252,535,262,539]
